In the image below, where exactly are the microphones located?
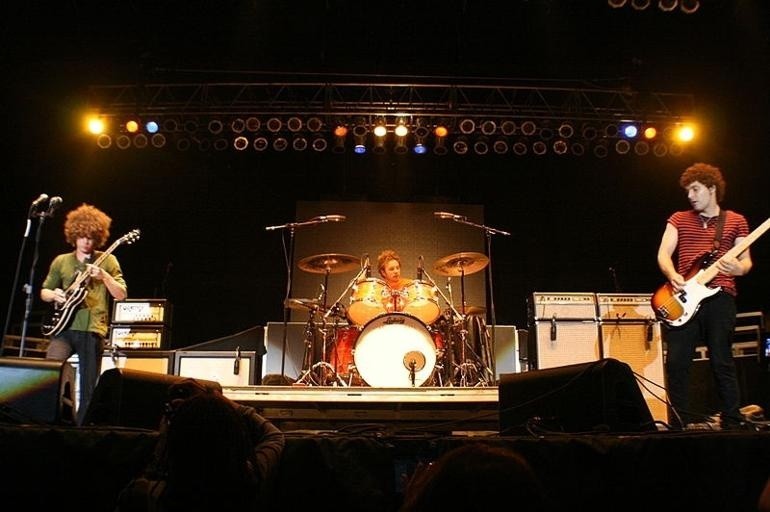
[366,266,370,278]
[326,216,346,221]
[31,193,49,209]
[433,211,463,219]
[49,197,63,215]
[417,266,422,280]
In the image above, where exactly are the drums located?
[352,312,437,387]
[348,277,394,327]
[396,280,441,325]
[324,327,364,386]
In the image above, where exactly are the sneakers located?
[721,418,757,427]
[670,412,687,432]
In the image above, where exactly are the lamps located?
[332,115,449,159]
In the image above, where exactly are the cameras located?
[163,389,186,423]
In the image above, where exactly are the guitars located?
[651,219,770,327]
[40,228,141,336]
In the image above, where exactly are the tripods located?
[265,219,368,387]
[423,217,510,388]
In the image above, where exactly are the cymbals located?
[298,254,360,274]
[449,306,487,316]
[284,298,318,313]
[431,252,489,277]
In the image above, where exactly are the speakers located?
[498,357,658,438]
[0,359,77,426]
[82,369,188,428]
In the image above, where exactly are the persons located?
[398,442,543,511]
[375,249,417,288]
[110,377,288,511]
[38,201,128,428]
[654,162,757,432]
